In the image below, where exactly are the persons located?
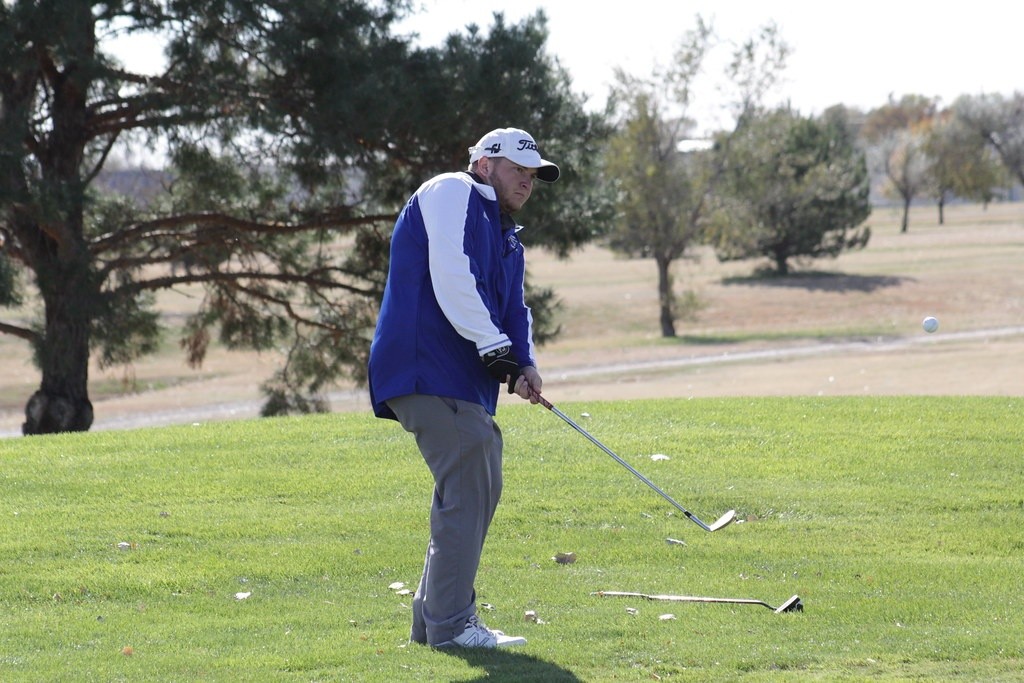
[368,128,541,651]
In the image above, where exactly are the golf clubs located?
[590,590,804,615]
[523,380,736,534]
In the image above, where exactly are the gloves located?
[485,347,521,394]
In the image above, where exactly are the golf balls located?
[922,317,940,333]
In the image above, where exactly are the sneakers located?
[432,619,527,649]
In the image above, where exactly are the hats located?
[470,127,560,184]
